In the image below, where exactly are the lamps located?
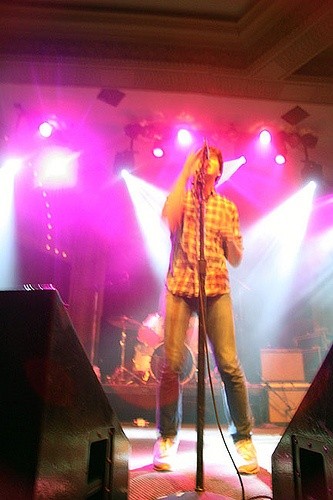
[301,134,322,190]
[114,124,144,177]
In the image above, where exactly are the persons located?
[153,147,260,475]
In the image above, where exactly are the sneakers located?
[235,438,258,474]
[154,435,178,471]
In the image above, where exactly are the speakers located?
[1,287,132,500]
[260,349,305,385]
[267,385,307,427]
[272,342,332,500]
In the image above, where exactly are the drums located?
[137,313,163,347]
[149,342,196,386]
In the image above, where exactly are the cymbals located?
[104,315,143,331]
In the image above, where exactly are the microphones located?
[202,146,208,162]
